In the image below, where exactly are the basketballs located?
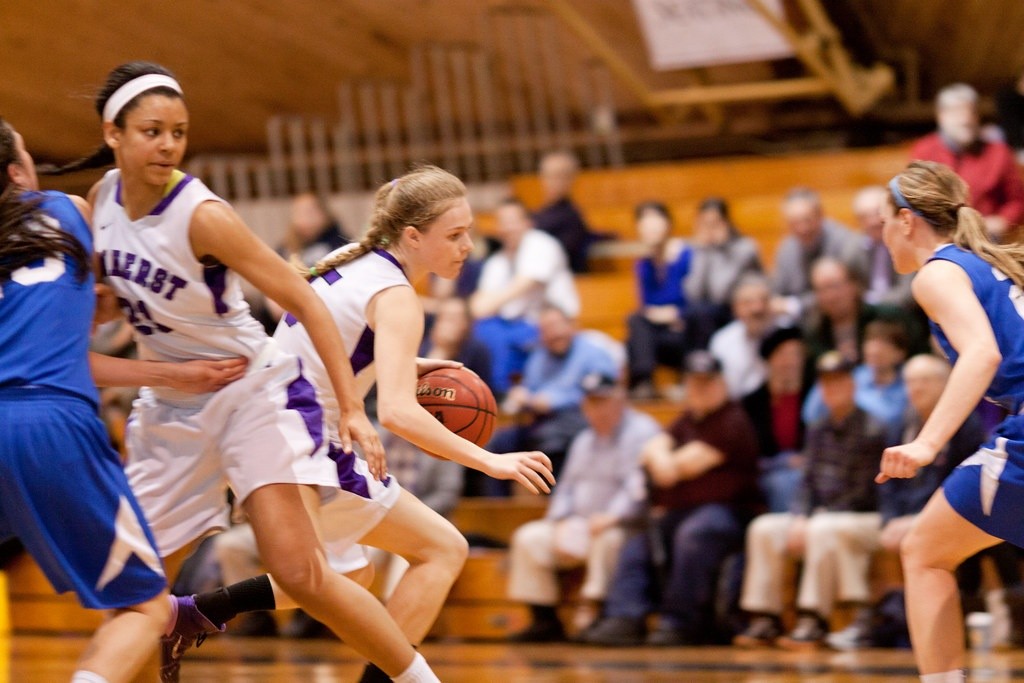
[417,368,498,461]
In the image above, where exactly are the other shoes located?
[503,601,1015,651]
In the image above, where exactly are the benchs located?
[424,150,921,641]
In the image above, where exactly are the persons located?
[241,167,557,683]
[0,113,178,683]
[85,59,440,683]
[876,156,1024,683]
[90,151,1024,652]
[912,81,1024,242]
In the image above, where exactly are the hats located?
[577,369,619,401]
[817,348,852,378]
[687,350,719,374]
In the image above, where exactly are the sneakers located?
[146,593,226,683]
